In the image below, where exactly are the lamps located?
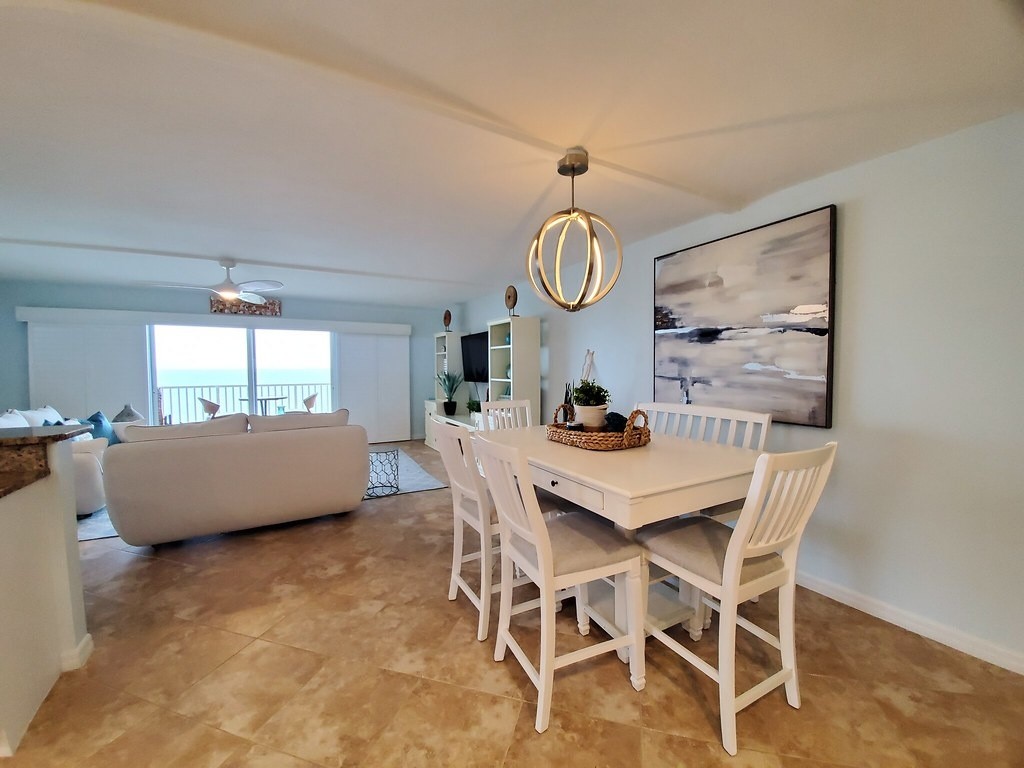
[527,147,623,315]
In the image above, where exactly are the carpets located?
[364,445,449,501]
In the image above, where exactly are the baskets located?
[545,404,651,450]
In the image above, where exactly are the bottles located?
[278,402,284,414]
[567,421,584,432]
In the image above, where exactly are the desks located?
[239,396,289,417]
[473,421,773,664]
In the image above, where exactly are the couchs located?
[101,424,369,546]
[0,402,144,515]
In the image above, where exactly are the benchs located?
[632,403,773,640]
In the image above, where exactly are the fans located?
[136,257,284,304]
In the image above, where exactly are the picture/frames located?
[653,204,837,428]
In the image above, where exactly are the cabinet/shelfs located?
[421,398,471,453]
[488,314,540,427]
[434,332,470,401]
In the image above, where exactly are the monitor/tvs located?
[461,331,489,383]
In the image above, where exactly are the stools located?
[365,444,400,498]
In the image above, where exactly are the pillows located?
[125,413,248,444]
[248,409,350,431]
[43,405,143,446]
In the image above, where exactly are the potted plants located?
[573,378,612,428]
[433,371,463,417]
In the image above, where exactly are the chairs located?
[284,391,319,416]
[198,397,231,419]
[431,402,839,756]
